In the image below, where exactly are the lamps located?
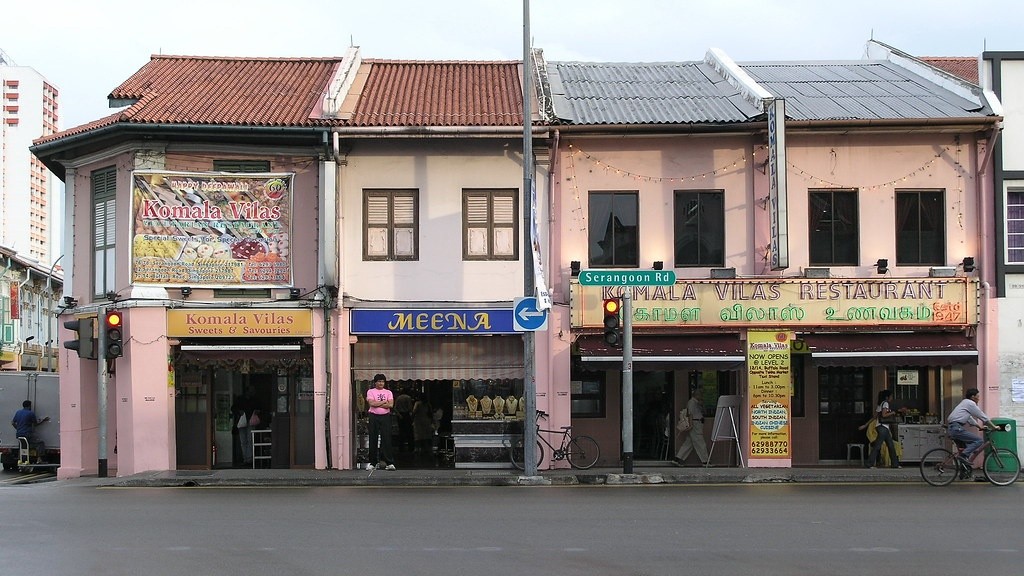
[106,292,120,305]
[290,288,300,300]
[180,286,192,299]
[877,259,889,276]
[653,262,664,270]
[571,260,581,276]
[963,257,975,272]
[63,296,79,308]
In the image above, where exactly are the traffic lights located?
[63,317,95,360]
[604,297,620,346]
[105,311,124,359]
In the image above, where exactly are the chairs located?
[846,420,867,469]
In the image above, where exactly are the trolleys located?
[16,436,61,475]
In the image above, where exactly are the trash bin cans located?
[983,417,1019,472]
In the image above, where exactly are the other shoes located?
[897,465,902,469]
[385,464,396,470]
[956,453,975,465]
[365,464,377,470]
[36,458,48,464]
[864,464,877,469]
[702,462,716,467]
[671,458,686,467]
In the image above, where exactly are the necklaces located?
[469,397,524,411]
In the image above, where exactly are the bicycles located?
[508,409,600,469]
[919,427,1022,488]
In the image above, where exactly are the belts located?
[692,418,702,421]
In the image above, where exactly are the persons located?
[859,390,905,468]
[366,374,396,470]
[12,400,50,463]
[947,388,1001,478]
[231,385,261,466]
[673,390,715,466]
[395,387,433,453]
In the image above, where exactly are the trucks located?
[0,370,62,470]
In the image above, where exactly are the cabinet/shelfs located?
[250,429,274,470]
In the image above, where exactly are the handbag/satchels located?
[676,407,692,433]
[878,401,897,424]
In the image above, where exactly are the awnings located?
[576,336,745,362]
[803,333,978,358]
